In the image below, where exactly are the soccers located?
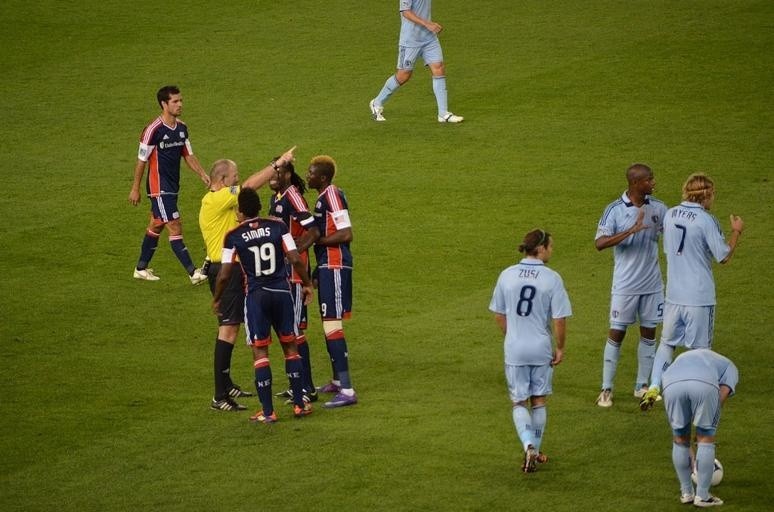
[691,458,722,486]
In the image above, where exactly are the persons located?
[662,348,739,508]
[269,155,321,406]
[594,163,670,408]
[638,172,745,411]
[305,155,358,410]
[211,187,314,424]
[368,0,465,123]
[487,228,573,473]
[127,85,212,287]
[198,144,297,413]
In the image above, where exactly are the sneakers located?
[368,99,386,121]
[679,491,696,505]
[315,382,342,394]
[227,384,254,398]
[247,410,278,424]
[521,445,550,473]
[694,494,724,507]
[324,389,359,408]
[292,402,313,417]
[595,387,615,408]
[132,267,161,282]
[437,112,464,123]
[210,396,248,412]
[189,267,209,287]
[275,388,318,405]
[634,386,663,411]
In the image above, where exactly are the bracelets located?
[269,159,281,172]
[732,229,741,235]
